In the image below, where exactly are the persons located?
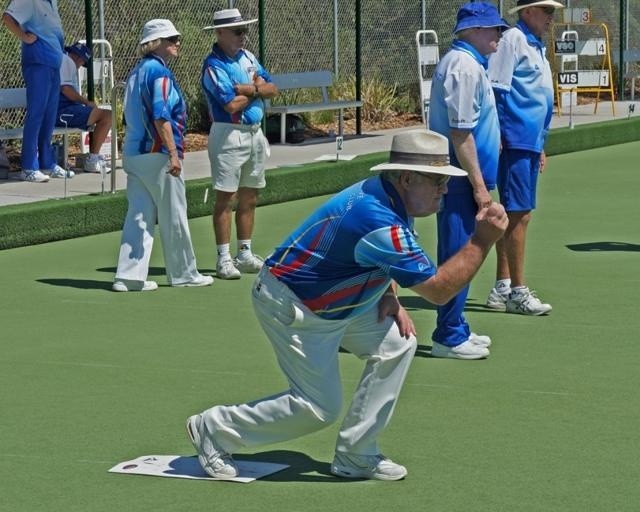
[428,1,510,357]
[54,43,111,172]
[487,1,565,318]
[111,19,214,291]
[3,0,74,183]
[186,129,508,482]
[202,7,278,278]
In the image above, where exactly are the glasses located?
[224,27,249,35]
[480,26,503,34]
[412,171,450,186]
[168,37,179,43]
[533,5,556,14]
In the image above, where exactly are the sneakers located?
[171,276,214,286]
[487,288,511,308]
[431,341,490,359]
[42,166,74,177]
[468,332,491,348]
[332,453,407,481]
[506,286,552,315]
[186,414,240,479]
[216,258,241,279]
[98,154,107,166]
[233,256,264,273]
[84,157,111,172]
[20,169,50,182]
[113,280,158,292]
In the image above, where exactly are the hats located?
[452,1,510,34]
[202,9,258,30]
[140,19,181,45]
[64,42,93,68]
[370,129,468,178]
[508,0,565,14]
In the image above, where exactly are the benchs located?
[257,71,362,143]
[0,87,96,173]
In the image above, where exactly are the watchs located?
[254,85,258,98]
